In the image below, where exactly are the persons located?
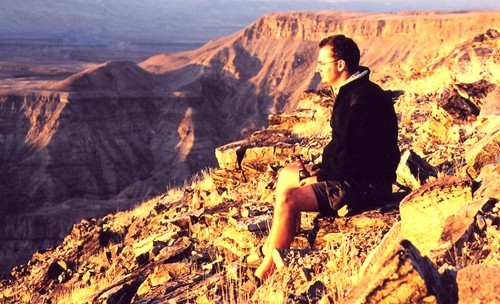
[236,34,402,297]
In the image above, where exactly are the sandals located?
[236,245,264,268]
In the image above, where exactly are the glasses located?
[319,59,340,65]
